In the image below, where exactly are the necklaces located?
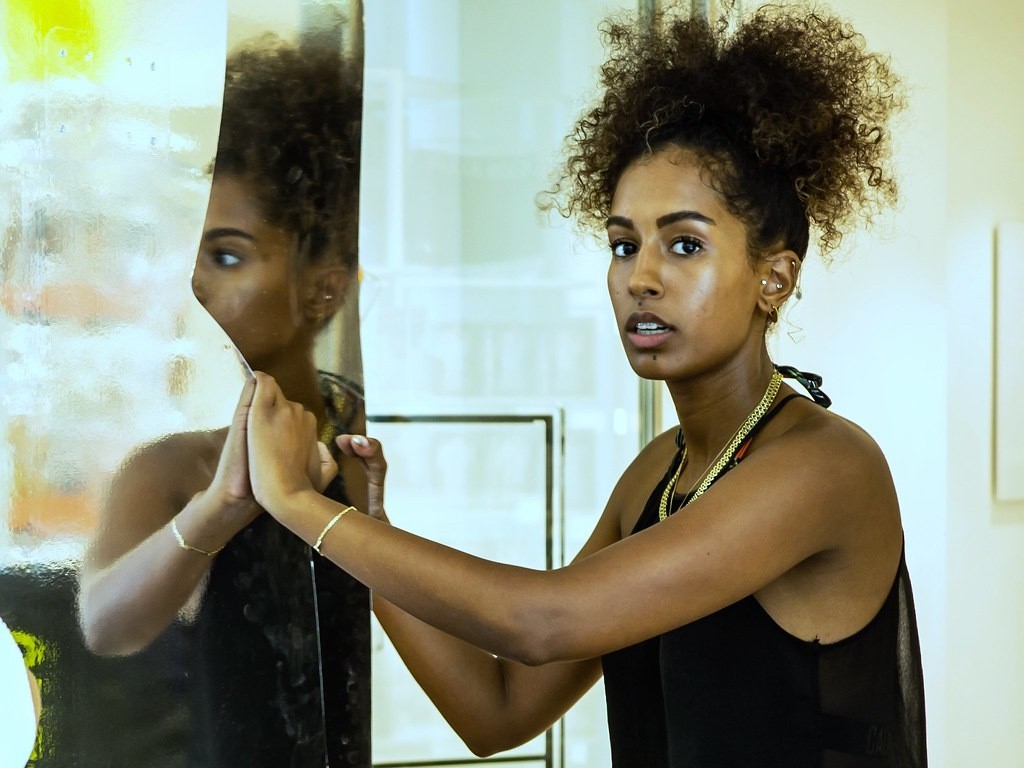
[658,369,782,523]
[320,394,347,446]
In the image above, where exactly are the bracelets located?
[313,507,357,554]
[167,517,227,557]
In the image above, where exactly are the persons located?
[247,2,927,768]
[77,40,372,767]
